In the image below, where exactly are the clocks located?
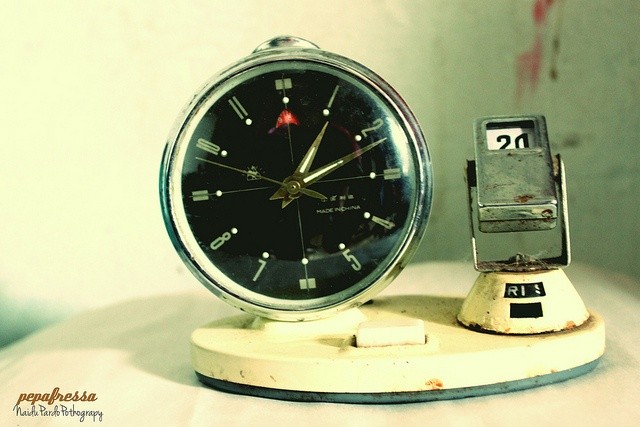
[154,34,438,325]
